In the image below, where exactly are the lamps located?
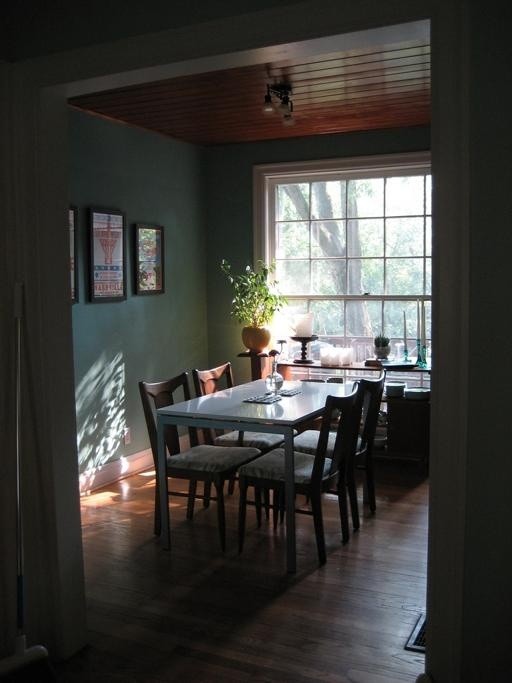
[262,81,300,129]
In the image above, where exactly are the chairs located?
[192,362,298,520]
[238,382,358,573]
[138,371,261,542]
[291,364,387,520]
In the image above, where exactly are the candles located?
[291,310,319,364]
[402,293,429,368]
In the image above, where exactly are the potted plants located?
[374,335,392,359]
[217,255,279,353]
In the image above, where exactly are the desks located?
[156,375,362,577]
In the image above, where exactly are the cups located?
[319,345,355,368]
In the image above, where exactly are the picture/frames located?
[66,204,81,306]
[88,202,128,303]
[131,221,168,294]
